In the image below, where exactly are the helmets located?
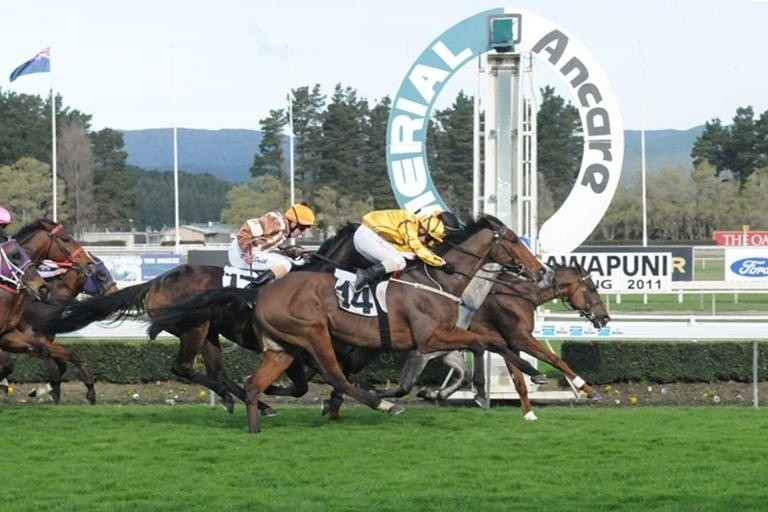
[414,211,460,244]
[285,204,317,228]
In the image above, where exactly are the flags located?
[9,44,50,84]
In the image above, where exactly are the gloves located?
[440,263,454,277]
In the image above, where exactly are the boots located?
[244,268,274,291]
[356,263,387,290]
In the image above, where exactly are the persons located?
[0,208,12,229]
[227,203,317,289]
[354,208,453,290]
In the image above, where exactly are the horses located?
[0,213,612,435]
